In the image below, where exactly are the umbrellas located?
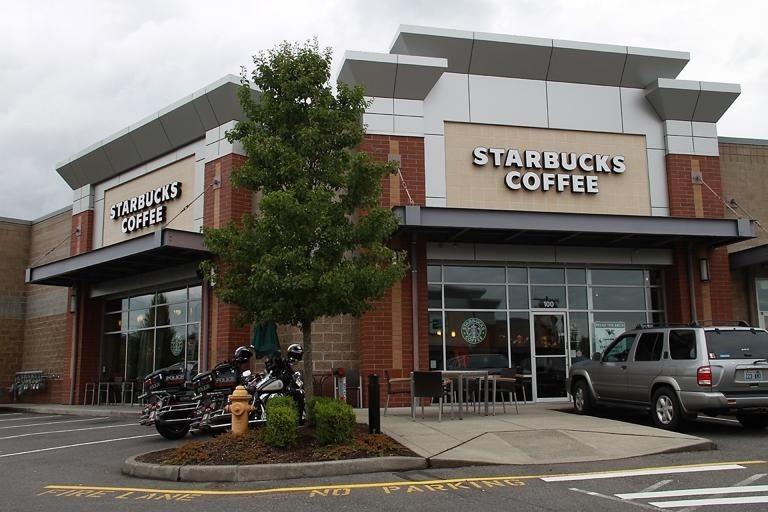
[250,319,281,361]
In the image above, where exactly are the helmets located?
[286,343,304,361]
[234,345,254,363]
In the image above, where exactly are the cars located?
[567,320,767,429]
[446,353,532,402]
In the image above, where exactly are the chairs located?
[383,367,520,422]
[84,381,146,405]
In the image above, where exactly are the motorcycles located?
[252,343,306,425]
[136,344,252,438]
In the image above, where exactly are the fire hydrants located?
[222,385,257,433]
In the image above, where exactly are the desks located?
[515,374,532,404]
